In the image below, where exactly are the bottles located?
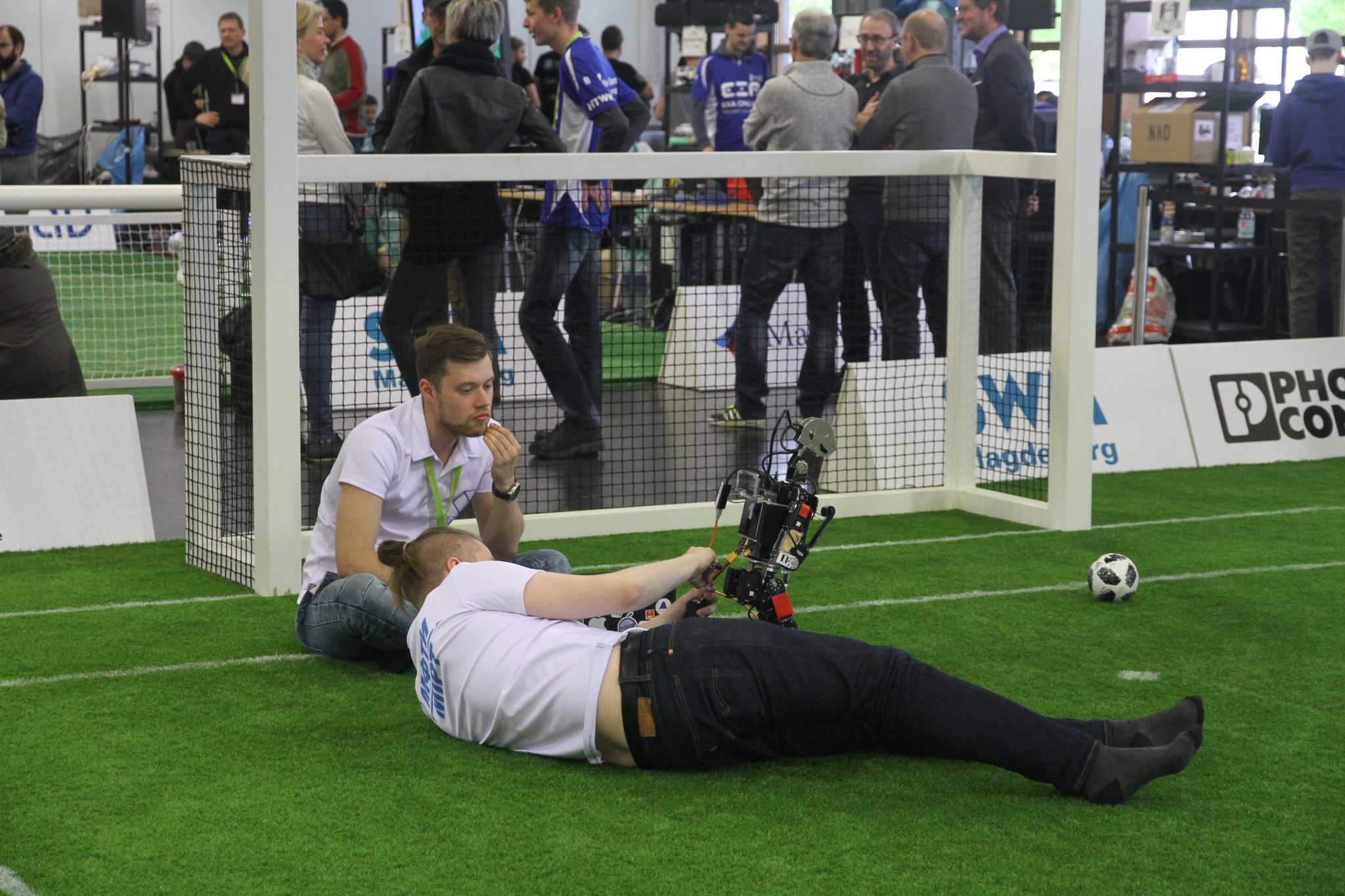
[1118,134,1131,162]
[1120,119,1132,137]
[1236,206,1256,248]
[1161,36,1180,75]
[1159,200,1176,246]
[1236,39,1252,83]
[1227,148,1235,164]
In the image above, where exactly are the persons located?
[374,527,1205,804]
[601,26,656,100]
[244,2,364,462]
[652,58,708,146]
[360,94,378,151]
[170,12,250,244]
[313,0,370,156]
[955,1,1041,355]
[832,11,903,395]
[372,0,568,408]
[501,36,541,108]
[518,1,651,457]
[709,9,858,429]
[691,5,774,205]
[859,9,977,362]
[1269,27,1345,337]
[161,40,209,142]
[533,22,592,119]
[1,224,85,399]
[295,322,573,670]
[0,24,44,231]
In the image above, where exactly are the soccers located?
[1085,550,1140,603]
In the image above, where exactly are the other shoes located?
[305,433,346,461]
[528,417,604,457]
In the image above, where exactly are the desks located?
[145,148,210,158]
[498,187,759,329]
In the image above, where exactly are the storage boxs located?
[1131,92,1267,164]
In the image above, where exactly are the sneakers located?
[708,404,768,427]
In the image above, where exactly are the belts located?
[621,629,645,768]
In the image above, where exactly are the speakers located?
[995,0,1055,29]
[101,0,146,39]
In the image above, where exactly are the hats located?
[423,0,452,9]
[184,40,205,62]
[1307,28,1343,54]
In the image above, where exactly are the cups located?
[1238,149,1255,165]
[1175,172,1232,197]
[1192,232,1205,243]
[1174,230,1192,243]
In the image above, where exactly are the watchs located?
[492,479,521,501]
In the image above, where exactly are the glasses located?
[955,4,981,15]
[894,35,905,49]
[425,11,445,23]
[856,34,893,44]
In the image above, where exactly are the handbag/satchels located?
[1106,264,1177,343]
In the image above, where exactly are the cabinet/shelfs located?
[664,22,773,151]
[1103,0,1291,343]
[79,25,162,184]
